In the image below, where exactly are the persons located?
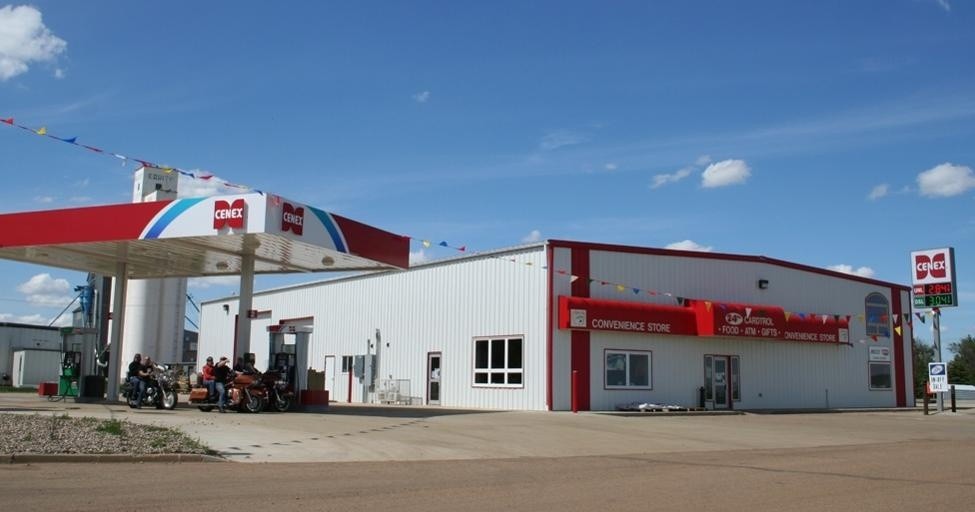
[244,353,256,375]
[135,356,165,409]
[235,358,243,371]
[203,357,217,400]
[129,354,143,400]
[214,357,240,413]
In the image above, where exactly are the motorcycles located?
[122,362,179,409]
[188,368,265,413]
[259,364,296,413]
